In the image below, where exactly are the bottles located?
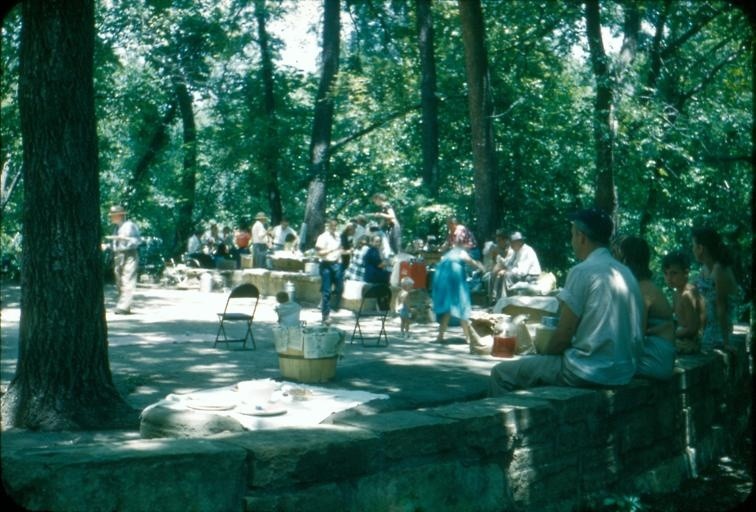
[491,314,516,357]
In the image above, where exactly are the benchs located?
[177,229,576,323]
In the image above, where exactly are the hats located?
[566,208,613,238]
[107,205,129,216]
[510,231,527,244]
[253,211,269,220]
[349,215,368,229]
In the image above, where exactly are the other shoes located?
[114,308,130,315]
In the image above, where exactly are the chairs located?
[350,282,391,348]
[213,282,260,352]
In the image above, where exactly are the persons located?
[273,292,301,328]
[692,230,737,352]
[489,208,644,398]
[661,251,706,355]
[101,205,142,316]
[316,193,402,326]
[620,237,676,385]
[189,213,305,270]
[397,216,543,354]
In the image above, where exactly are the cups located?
[542,317,558,329]
